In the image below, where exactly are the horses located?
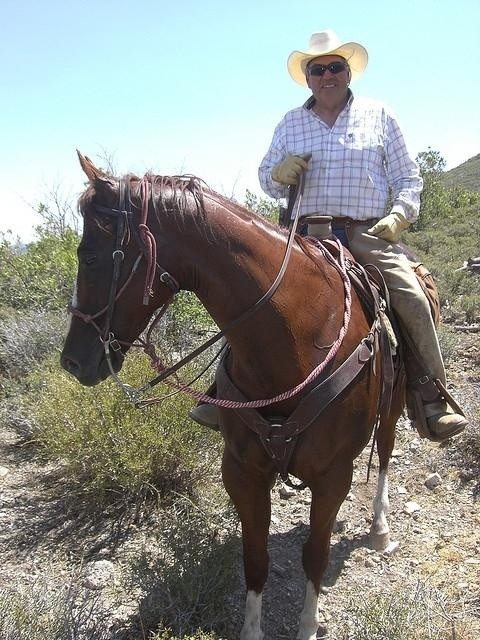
[59,147,443,640]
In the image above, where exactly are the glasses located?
[309,61,345,76]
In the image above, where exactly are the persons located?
[190,55,469,438]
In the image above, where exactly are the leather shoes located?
[187,404,221,429]
[427,413,469,438]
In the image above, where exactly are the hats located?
[287,31,369,86]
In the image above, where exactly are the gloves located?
[271,151,312,185]
[368,214,410,240]
